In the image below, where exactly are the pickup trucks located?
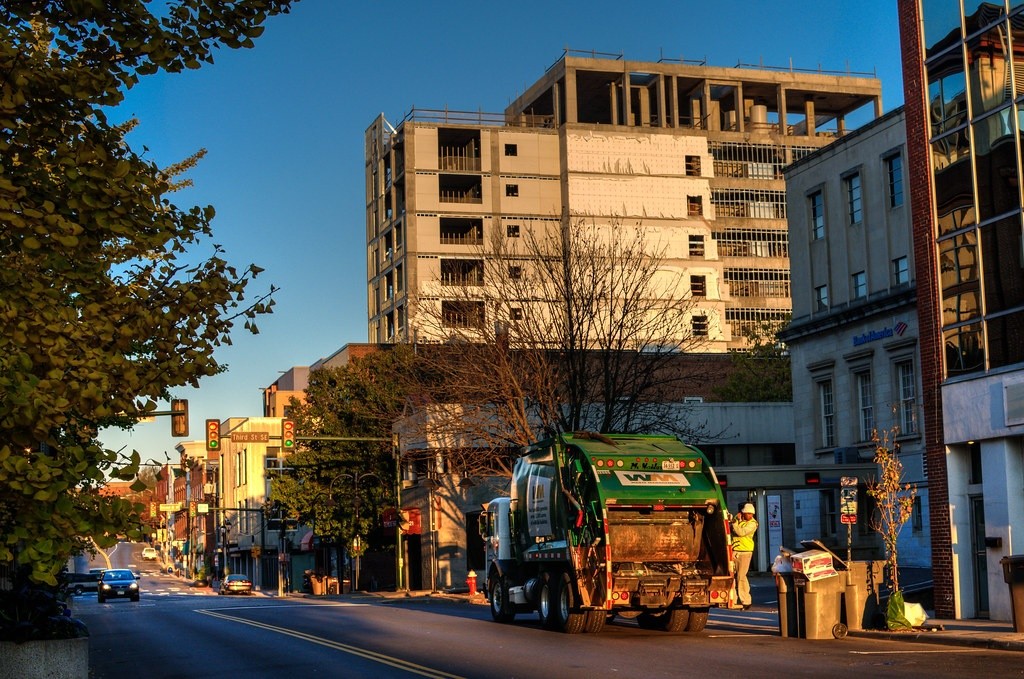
[142,548,158,560]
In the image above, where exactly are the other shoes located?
[740,604,751,611]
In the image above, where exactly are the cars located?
[219,574,252,595]
[98,569,140,603]
[89,568,107,573]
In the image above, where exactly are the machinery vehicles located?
[478,425,743,635]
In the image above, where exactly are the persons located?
[728,503,759,612]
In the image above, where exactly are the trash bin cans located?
[843,559,888,630]
[309,575,327,595]
[774,571,798,638]
[795,540,849,640]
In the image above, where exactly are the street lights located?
[220,519,232,568]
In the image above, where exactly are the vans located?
[66,572,102,595]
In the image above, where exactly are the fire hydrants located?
[465,569,477,595]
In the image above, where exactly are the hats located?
[740,503,756,515]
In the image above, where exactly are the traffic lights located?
[282,419,295,452]
[150,502,156,517]
[206,419,221,451]
[190,501,196,518]
